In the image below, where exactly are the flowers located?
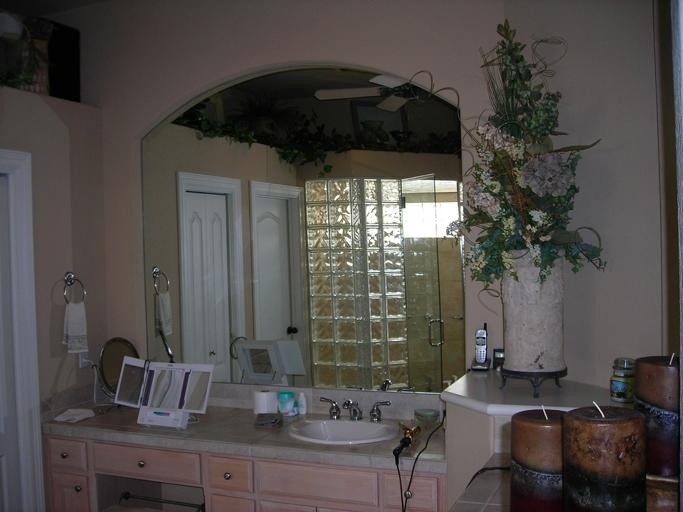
[445,17,607,292]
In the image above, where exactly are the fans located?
[314,74,427,113]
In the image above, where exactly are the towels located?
[54,408,95,423]
[153,291,173,338]
[62,301,90,353]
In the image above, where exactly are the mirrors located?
[114,355,216,415]
[97,336,140,397]
[140,60,467,395]
[237,339,305,386]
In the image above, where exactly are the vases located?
[500,246,568,397]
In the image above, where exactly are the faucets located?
[343,398,362,421]
[382,379,391,391]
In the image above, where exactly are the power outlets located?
[79,351,90,369]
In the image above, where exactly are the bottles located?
[278,392,299,417]
[610,358,635,403]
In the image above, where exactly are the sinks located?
[290,414,400,444]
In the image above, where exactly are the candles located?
[510,354,681,512]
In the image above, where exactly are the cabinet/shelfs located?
[42,433,447,512]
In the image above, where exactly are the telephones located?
[471,323,491,371]
